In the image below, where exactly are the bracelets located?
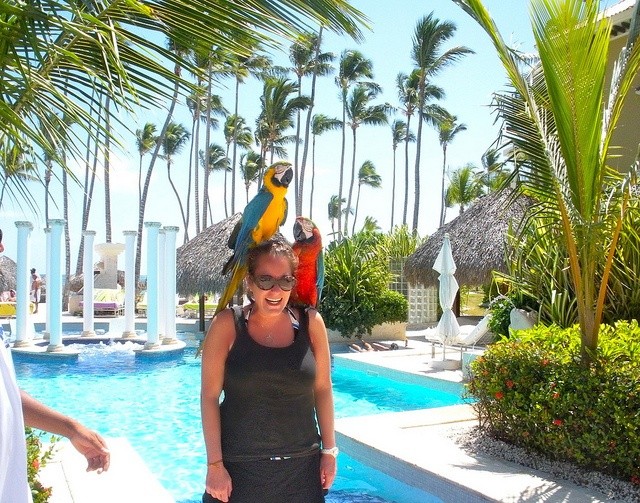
[207,458,223,468]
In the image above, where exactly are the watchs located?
[320,446,339,458]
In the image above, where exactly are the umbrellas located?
[432,231,461,372]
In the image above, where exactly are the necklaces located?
[251,311,285,344]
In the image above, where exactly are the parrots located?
[195,160,293,358]
[285,216,324,317]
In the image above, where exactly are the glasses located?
[248,269,297,291]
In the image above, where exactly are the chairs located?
[424,311,496,367]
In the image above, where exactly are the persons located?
[199,240,339,503]
[5,289,16,302]
[29,274,39,313]
[29,267,42,291]
[0,227,111,503]
